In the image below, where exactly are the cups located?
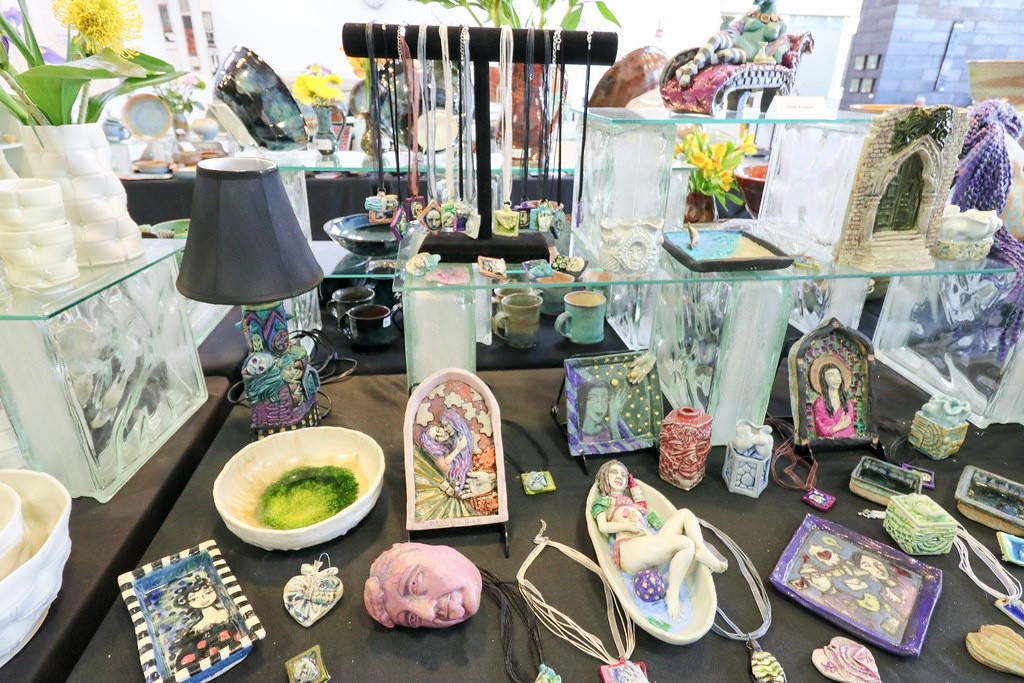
[339,304,392,352]
[530,271,576,317]
[494,282,533,330]
[326,286,375,328]
[554,290,607,346]
[493,293,544,349]
[579,266,615,318]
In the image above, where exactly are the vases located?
[311,105,346,155]
[0,178,81,292]
[359,111,389,155]
[721,417,774,500]
[21,124,143,269]
[661,405,713,490]
[172,111,189,141]
[497,59,569,161]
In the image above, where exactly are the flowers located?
[0,1,192,125]
[292,65,343,106]
[675,124,757,213]
[339,46,393,110]
[152,73,206,113]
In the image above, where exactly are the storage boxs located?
[848,456,924,505]
[878,494,958,555]
[956,464,1024,537]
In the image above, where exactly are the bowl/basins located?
[323,212,401,257]
[213,426,385,551]
[207,43,310,151]
[0,468,73,668]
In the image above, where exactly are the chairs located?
[660,32,814,115]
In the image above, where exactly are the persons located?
[294,657,318,681]
[813,363,856,437]
[591,460,728,623]
[363,542,483,628]
[439,410,472,495]
[421,424,492,499]
[675,0,790,89]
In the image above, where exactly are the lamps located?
[175,156,325,442]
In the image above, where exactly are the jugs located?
[102,116,131,142]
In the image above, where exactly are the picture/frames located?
[551,347,663,473]
[788,316,882,452]
[996,531,1024,567]
[901,462,936,489]
[771,512,945,657]
[117,538,267,683]
[402,367,513,560]
[281,645,332,683]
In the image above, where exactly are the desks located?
[565,105,874,255]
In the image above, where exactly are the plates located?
[170,164,197,180]
[122,93,172,141]
[135,160,171,175]
